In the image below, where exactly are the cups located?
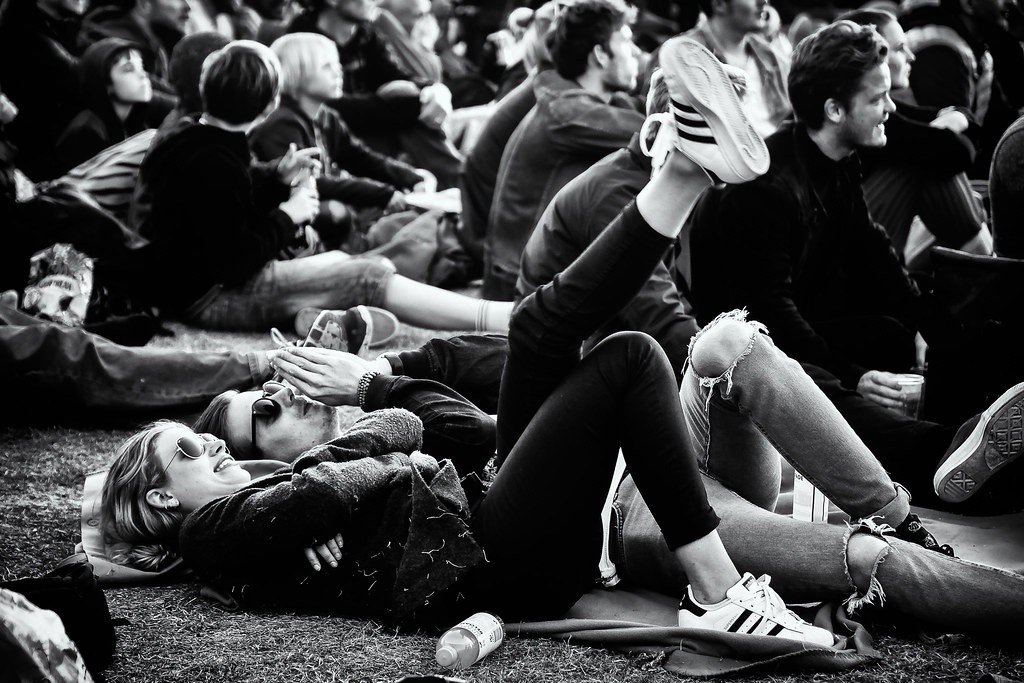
[883,373,924,420]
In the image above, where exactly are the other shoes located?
[933,382,1024,503]
[896,513,954,558]
[270,305,346,353]
[297,306,398,346]
[346,306,373,360]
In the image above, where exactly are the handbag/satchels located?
[375,211,482,290]
[57,467,185,587]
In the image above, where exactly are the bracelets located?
[357,371,380,406]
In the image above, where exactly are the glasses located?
[252,381,286,452]
[158,433,217,481]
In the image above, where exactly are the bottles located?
[289,162,317,221]
[436,611,507,670]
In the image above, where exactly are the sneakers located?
[677,571,833,647]
[638,37,770,189]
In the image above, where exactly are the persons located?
[0,0,1024,649]
